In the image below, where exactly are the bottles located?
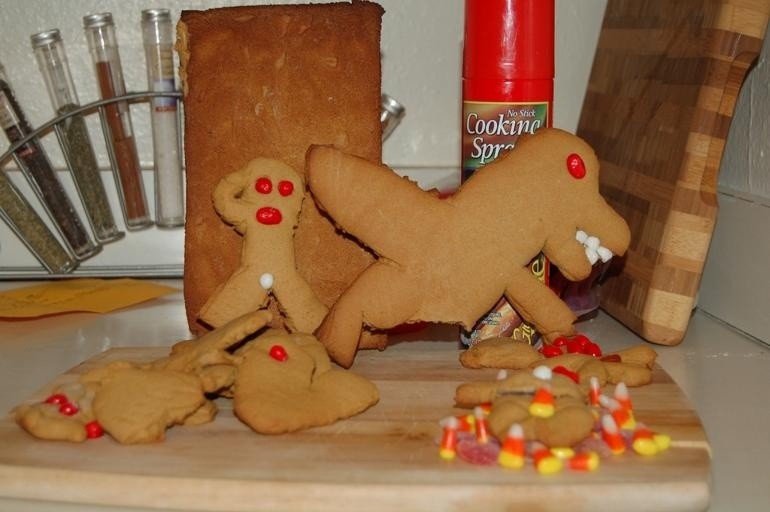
[31,28,125,245]
[84,10,153,231]
[1,65,103,261]
[458,0,556,351]
[140,7,186,229]
[1,170,79,275]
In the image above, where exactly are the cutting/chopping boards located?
[574,1,769,347]
[1,346,714,511]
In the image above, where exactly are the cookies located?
[178,1,389,351]
[16,127,658,448]
